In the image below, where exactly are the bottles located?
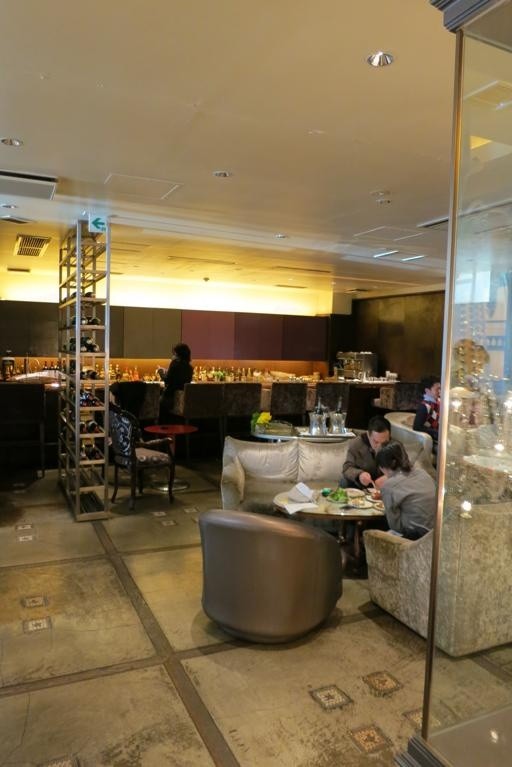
[50,360,55,370]
[133,366,139,381]
[69,336,93,346]
[123,368,128,381]
[64,291,84,303]
[88,343,100,352]
[62,360,66,372]
[83,389,94,401]
[247,368,252,383]
[94,364,122,382]
[56,361,60,370]
[44,360,48,371]
[80,397,87,406]
[71,316,88,325]
[128,370,133,382]
[241,368,247,383]
[65,343,87,352]
[80,438,97,459]
[73,421,87,434]
[80,372,85,379]
[92,397,100,406]
[6,365,24,378]
[89,316,101,325]
[87,419,100,433]
[87,370,99,379]
[85,292,96,298]
[192,366,240,384]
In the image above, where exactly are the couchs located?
[198,508,342,645]
[383,411,439,480]
[219,435,423,536]
[364,500,512,656]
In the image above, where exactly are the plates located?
[344,488,365,497]
[373,502,385,510]
[367,487,381,493]
[347,498,373,509]
[365,495,382,503]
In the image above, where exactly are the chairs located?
[105,405,173,512]
[168,383,225,459]
[367,381,421,431]
[225,383,262,453]
[262,383,307,426]
[3,383,47,480]
[305,383,349,429]
[109,381,160,462]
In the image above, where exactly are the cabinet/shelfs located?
[57,218,110,522]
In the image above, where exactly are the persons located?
[158,344,193,457]
[351,441,437,579]
[414,377,442,466]
[338,419,390,549]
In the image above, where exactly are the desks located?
[145,425,197,491]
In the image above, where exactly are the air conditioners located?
[0,169,59,200]
